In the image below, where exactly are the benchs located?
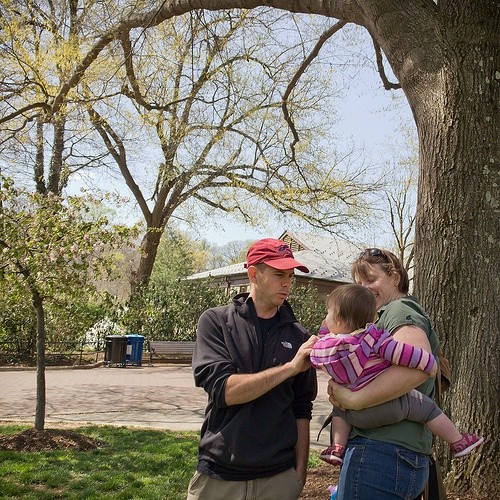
[146,340,196,365]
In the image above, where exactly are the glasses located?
[358,248,390,264]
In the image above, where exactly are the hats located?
[244,237,310,273]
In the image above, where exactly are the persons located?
[310,284,484,466]
[187,238,318,500]
[327,249,441,500]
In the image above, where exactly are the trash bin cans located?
[125,335,144,367]
[104,335,128,368]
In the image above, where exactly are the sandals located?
[319,445,345,466]
[447,433,484,457]
